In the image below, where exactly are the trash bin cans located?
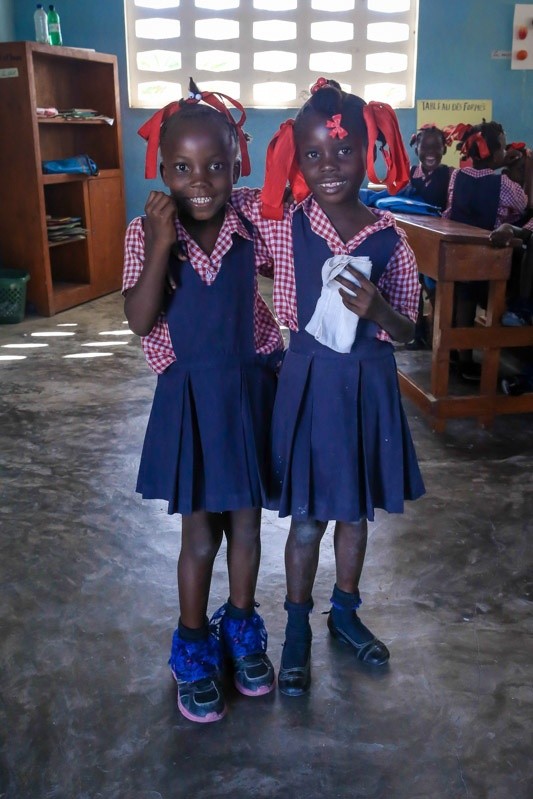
[0,269,30,324]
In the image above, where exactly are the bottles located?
[47,5,63,47]
[34,4,50,45]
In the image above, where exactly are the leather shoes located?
[327,615,390,666]
[277,641,311,697]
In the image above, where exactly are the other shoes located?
[448,362,460,374]
[502,379,528,396]
[407,338,427,350]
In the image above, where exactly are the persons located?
[120,78,279,724]
[230,77,426,695]
[401,117,533,396]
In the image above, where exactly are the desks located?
[384,211,523,431]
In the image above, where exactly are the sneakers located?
[219,613,276,697]
[168,628,228,723]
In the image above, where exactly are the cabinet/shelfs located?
[0,41,127,316]
